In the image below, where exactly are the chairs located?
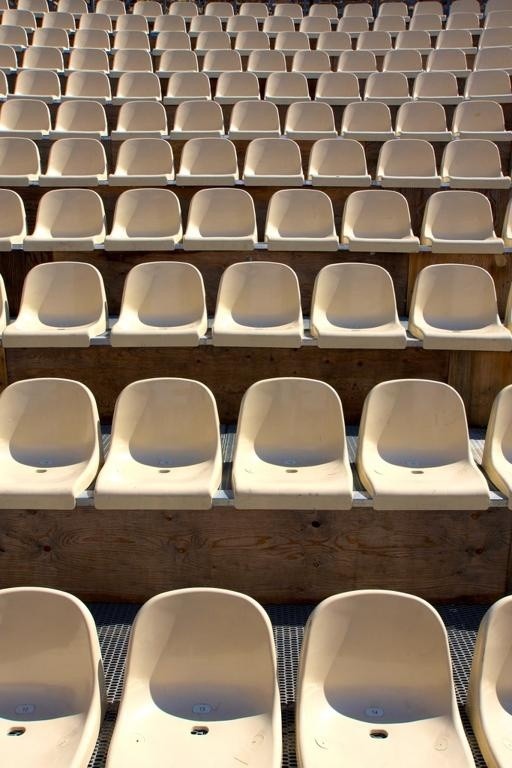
[408,13,443,37]
[0,377,105,511]
[13,70,61,103]
[193,31,232,57]
[0,24,29,52]
[175,137,239,187]
[57,0,89,19]
[0,44,18,75]
[93,377,222,511]
[95,0,127,21]
[73,28,111,54]
[298,16,332,39]
[204,2,234,23]
[77,12,114,34]
[0,188,27,252]
[153,32,192,56]
[213,71,261,104]
[163,71,212,106]
[336,50,380,79]
[373,15,406,38]
[0,98,52,140]
[451,100,512,142]
[412,1,447,22]
[111,30,151,53]
[112,72,163,106]
[444,11,483,35]
[233,30,270,56]
[64,48,110,76]
[110,260,208,347]
[473,47,512,75]
[111,100,168,141]
[478,28,512,47]
[395,100,452,142]
[104,587,284,768]
[434,29,477,55]
[314,72,362,105]
[110,49,154,79]
[413,71,464,105]
[316,31,352,57]
[0,136,42,187]
[241,137,305,187]
[382,50,423,79]
[18,46,64,74]
[155,49,200,79]
[294,588,476,767]
[355,378,491,511]
[151,14,187,33]
[2,261,108,348]
[211,260,304,349]
[464,69,512,103]
[263,15,296,39]
[41,11,76,36]
[284,101,338,139]
[355,30,394,56]
[62,71,112,105]
[264,188,339,252]
[246,50,288,79]
[449,0,483,20]
[377,2,411,23]
[182,187,258,251]
[466,594,512,768]
[343,3,375,23]
[200,49,243,78]
[239,2,269,24]
[132,0,164,22]
[48,100,109,142]
[31,27,70,54]
[363,72,413,106]
[104,187,183,252]
[340,189,420,254]
[112,14,149,35]
[263,72,312,105]
[274,31,311,56]
[483,0,512,16]
[291,49,334,79]
[38,137,108,187]
[425,48,472,78]
[308,3,340,24]
[340,101,396,142]
[482,384,512,512]
[1,9,37,34]
[483,10,512,28]
[395,29,432,55]
[375,138,442,189]
[226,15,259,38]
[274,3,304,24]
[170,99,225,141]
[227,99,282,141]
[168,1,199,22]
[336,16,370,39]
[22,188,107,252]
[188,15,223,38]
[230,376,354,511]
[0,586,106,768]
[307,138,372,188]
[16,0,49,18]
[407,262,512,352]
[309,262,407,350]
[440,138,512,190]
[420,190,505,255]
[108,137,176,186]
